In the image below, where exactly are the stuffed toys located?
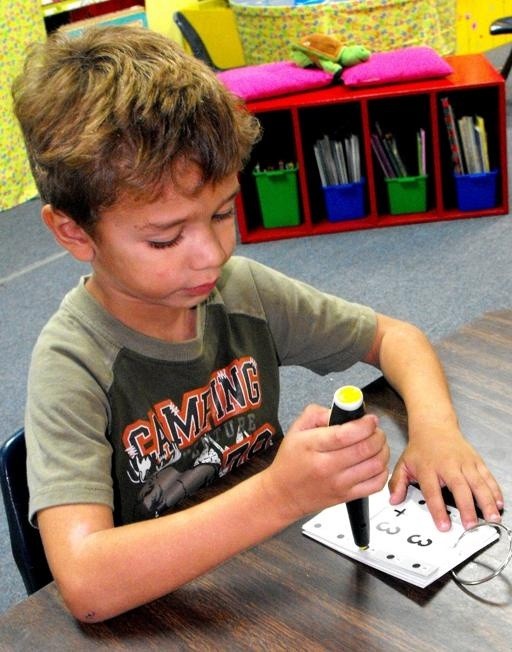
[289,33,372,77]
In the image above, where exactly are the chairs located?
[1,425,55,597]
[488,14,512,82]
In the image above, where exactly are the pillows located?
[216,60,335,103]
[339,45,455,89]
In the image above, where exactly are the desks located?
[1,303,512,652]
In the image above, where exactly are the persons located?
[11,25,506,629]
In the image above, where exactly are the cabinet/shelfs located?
[229,52,509,243]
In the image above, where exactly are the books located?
[253,94,493,181]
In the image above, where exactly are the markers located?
[329,385,370,550]
